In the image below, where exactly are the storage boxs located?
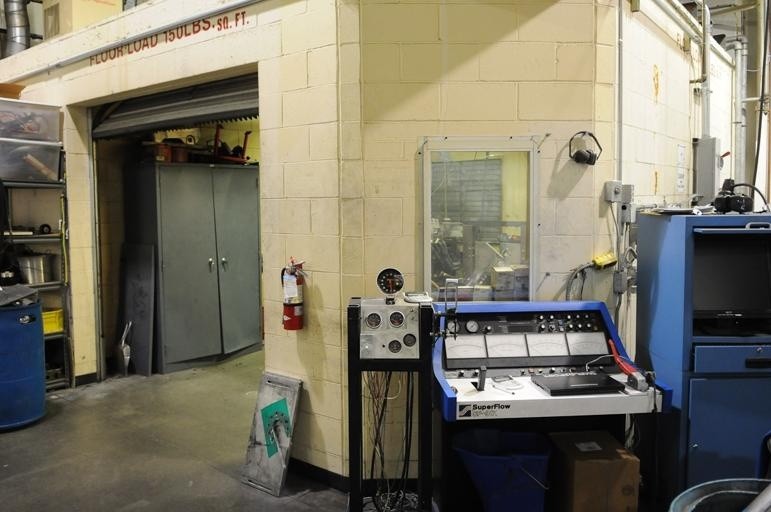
[0,98,62,183]
[545,430,640,512]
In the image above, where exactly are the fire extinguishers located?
[282,257,307,330]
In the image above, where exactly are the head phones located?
[568,130,604,167]
[713,189,754,212]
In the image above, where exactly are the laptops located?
[531,371,625,397]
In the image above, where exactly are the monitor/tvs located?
[694,232,770,337]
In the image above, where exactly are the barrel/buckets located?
[0,296,50,433]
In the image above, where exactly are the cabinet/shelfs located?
[635,210,770,512]
[120,160,262,375]
[0,182,70,392]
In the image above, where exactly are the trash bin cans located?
[0,286,47,432]
[463,432,553,511]
